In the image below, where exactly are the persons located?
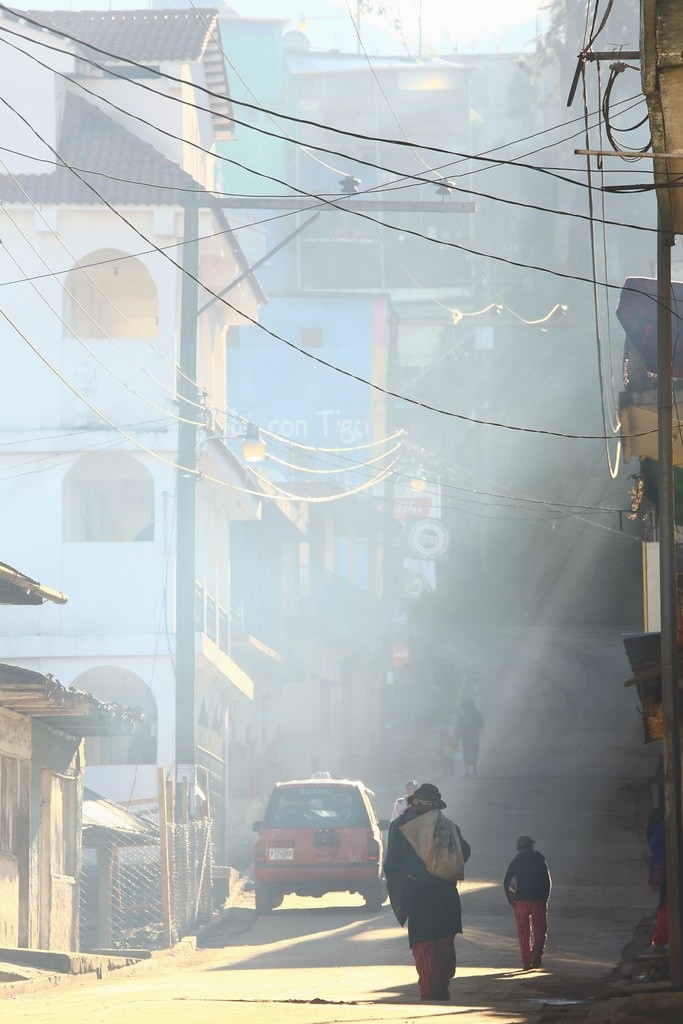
[647,805,670,951]
[390,700,484,818]
[383,784,474,1002]
[505,836,553,968]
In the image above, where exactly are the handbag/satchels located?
[648,857,666,885]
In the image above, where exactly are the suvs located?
[250,772,392,912]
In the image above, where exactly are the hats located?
[407,783,446,811]
[516,836,536,850]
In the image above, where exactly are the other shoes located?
[532,951,541,970]
[438,978,450,1000]
[652,938,666,955]
[523,963,531,970]
